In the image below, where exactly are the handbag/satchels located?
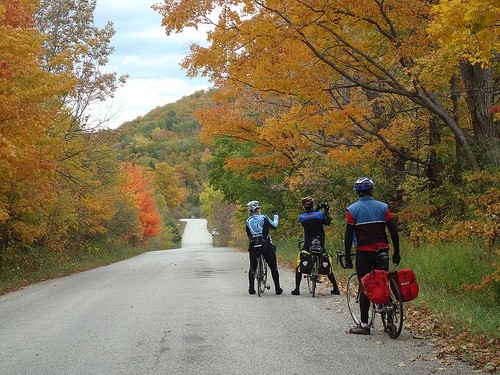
[389,269,419,302]
[296,250,312,274]
[360,269,390,303]
[318,253,333,274]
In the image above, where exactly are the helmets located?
[354,177,374,193]
[301,197,314,207]
[246,200,261,210]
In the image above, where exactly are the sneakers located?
[349,324,371,335]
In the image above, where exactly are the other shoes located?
[249,288,256,294]
[291,289,300,295]
[331,288,340,295]
[276,287,283,295]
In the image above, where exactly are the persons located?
[245,200,283,295]
[345,177,401,335]
[291,197,340,296]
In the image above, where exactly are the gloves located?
[345,257,353,269]
[316,202,330,211]
[272,210,279,215]
[391,253,401,265]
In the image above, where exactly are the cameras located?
[319,203,325,207]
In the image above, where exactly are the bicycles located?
[297,236,323,297]
[255,235,276,297]
[336,250,404,339]
